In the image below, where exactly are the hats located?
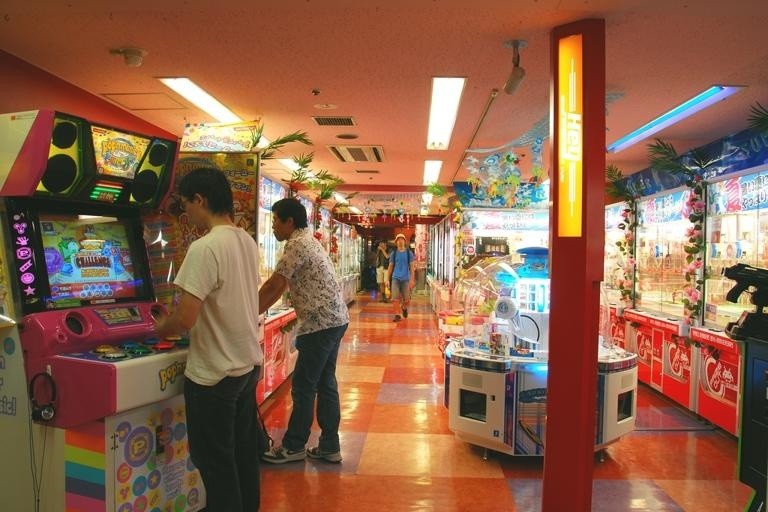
[394,233,406,243]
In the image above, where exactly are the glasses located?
[179,199,191,210]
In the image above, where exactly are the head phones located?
[30,373,56,421]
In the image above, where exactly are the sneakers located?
[403,308,408,317]
[261,446,342,463]
[393,315,401,321]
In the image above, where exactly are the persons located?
[376,240,392,303]
[259,199,350,464]
[387,233,415,322]
[158,168,263,512]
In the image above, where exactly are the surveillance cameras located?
[504,67,525,95]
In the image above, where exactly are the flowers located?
[647,138,706,327]
[604,164,638,309]
[282,153,339,264]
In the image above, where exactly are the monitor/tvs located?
[36,212,148,304]
